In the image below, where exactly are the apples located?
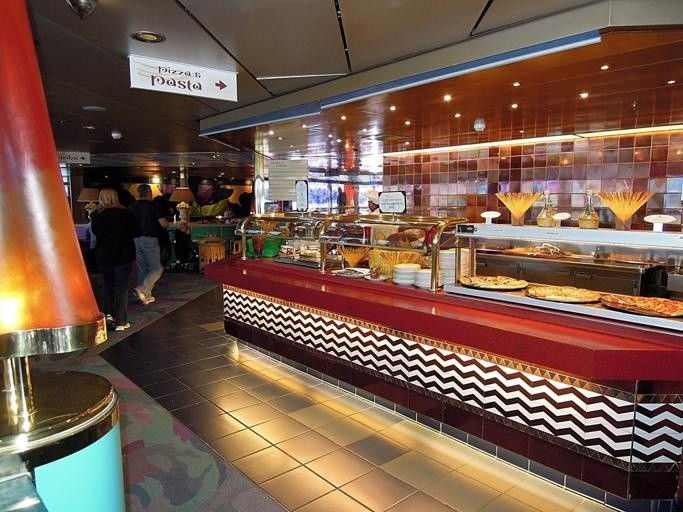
[371,271,378,279]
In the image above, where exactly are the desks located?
[74,223,98,249]
[168,221,242,261]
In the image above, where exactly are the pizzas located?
[602,290,683,317]
[460,274,525,289]
[525,284,602,304]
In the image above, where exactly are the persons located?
[89,186,141,331]
[364,191,380,214]
[125,184,165,308]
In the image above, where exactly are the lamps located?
[0,0,127,512]
[112,133,122,140]
[76,187,100,204]
[168,186,196,202]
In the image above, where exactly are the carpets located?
[29,261,290,512]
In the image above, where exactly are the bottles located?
[537,190,556,227]
[576,190,600,229]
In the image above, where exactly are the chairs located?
[172,228,199,274]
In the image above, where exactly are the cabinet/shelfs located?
[235,211,360,269]
[317,213,470,293]
[443,222,683,333]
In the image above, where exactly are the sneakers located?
[115,322,131,331]
[106,314,115,328]
[130,287,156,307]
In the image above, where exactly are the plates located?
[437,248,476,284]
[415,268,442,289]
[363,274,389,282]
[392,263,422,286]
